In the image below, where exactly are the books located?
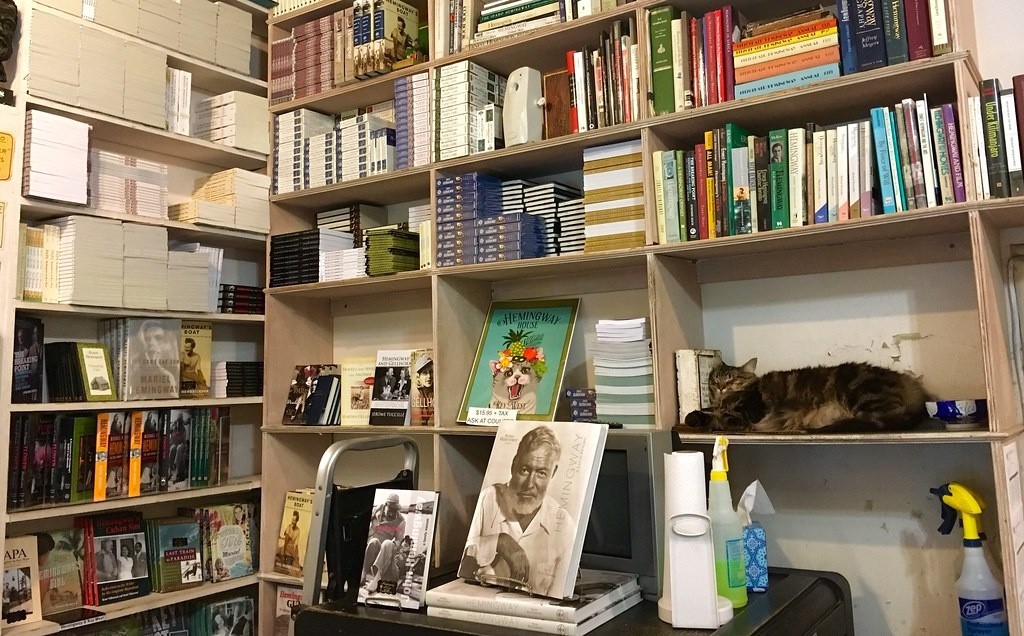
[0,0,316,636]
[337,349,435,428]
[425,314,722,635]
[356,487,441,610]
[280,363,341,425]
[266,0,1023,291]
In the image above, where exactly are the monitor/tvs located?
[580,429,672,602]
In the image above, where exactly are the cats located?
[686,350,931,430]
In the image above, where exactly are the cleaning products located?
[706,434,748,608]
[930,482,1009,636]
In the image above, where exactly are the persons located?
[413,356,435,426]
[397,367,410,398]
[0,0,18,84]
[390,16,407,61]
[381,367,397,402]
[0,318,412,636]
[288,367,319,422]
[457,422,575,598]
[770,143,783,163]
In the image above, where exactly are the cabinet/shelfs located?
[0,0,1024,636]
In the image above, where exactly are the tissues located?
[734,479,776,589]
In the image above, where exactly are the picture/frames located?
[454,296,582,423]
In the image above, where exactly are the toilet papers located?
[662,449,707,608]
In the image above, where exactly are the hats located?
[415,355,432,373]
[387,494,402,510]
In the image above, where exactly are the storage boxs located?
[543,70,572,139]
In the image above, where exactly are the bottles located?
[709,435,748,608]
[928,483,1011,636]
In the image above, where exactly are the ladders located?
[284,434,421,635]
